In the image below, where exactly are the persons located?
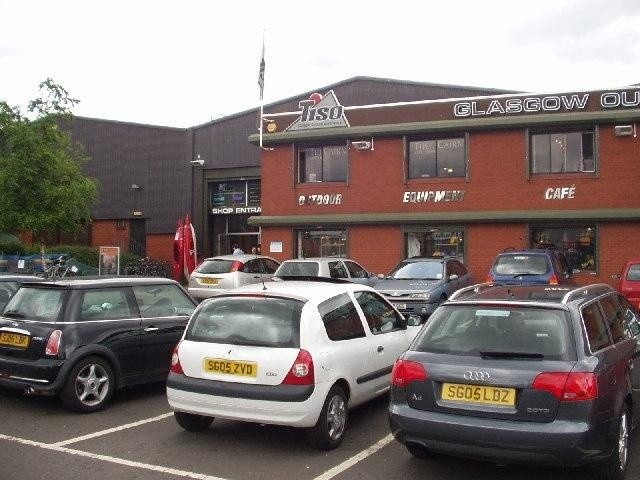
[231,242,245,256]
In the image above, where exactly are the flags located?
[257,41,265,100]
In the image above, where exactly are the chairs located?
[441,313,566,369]
[22,295,172,336]
[213,308,359,354]
